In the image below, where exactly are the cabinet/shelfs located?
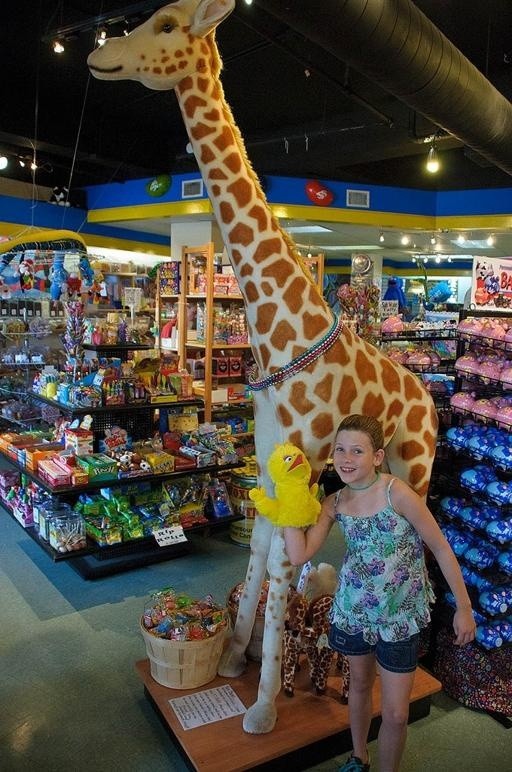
[381,329,458,503]
[0,308,62,430]
[0,344,246,582]
[433,309,512,730]
[179,242,325,538]
[155,269,180,359]
[83,272,153,344]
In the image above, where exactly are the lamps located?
[431,232,436,245]
[379,225,384,242]
[426,139,440,172]
[97,22,109,44]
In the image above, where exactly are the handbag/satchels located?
[433,622,512,716]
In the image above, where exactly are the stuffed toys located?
[0,250,104,298]
[382,276,403,310]
[253,582,350,705]
[249,441,321,527]
[84,0,439,731]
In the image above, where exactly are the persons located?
[282,414,476,771]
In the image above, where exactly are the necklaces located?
[347,473,381,491]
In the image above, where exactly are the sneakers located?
[341,742,371,772]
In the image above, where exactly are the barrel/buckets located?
[140,601,231,691]
[228,607,266,663]
